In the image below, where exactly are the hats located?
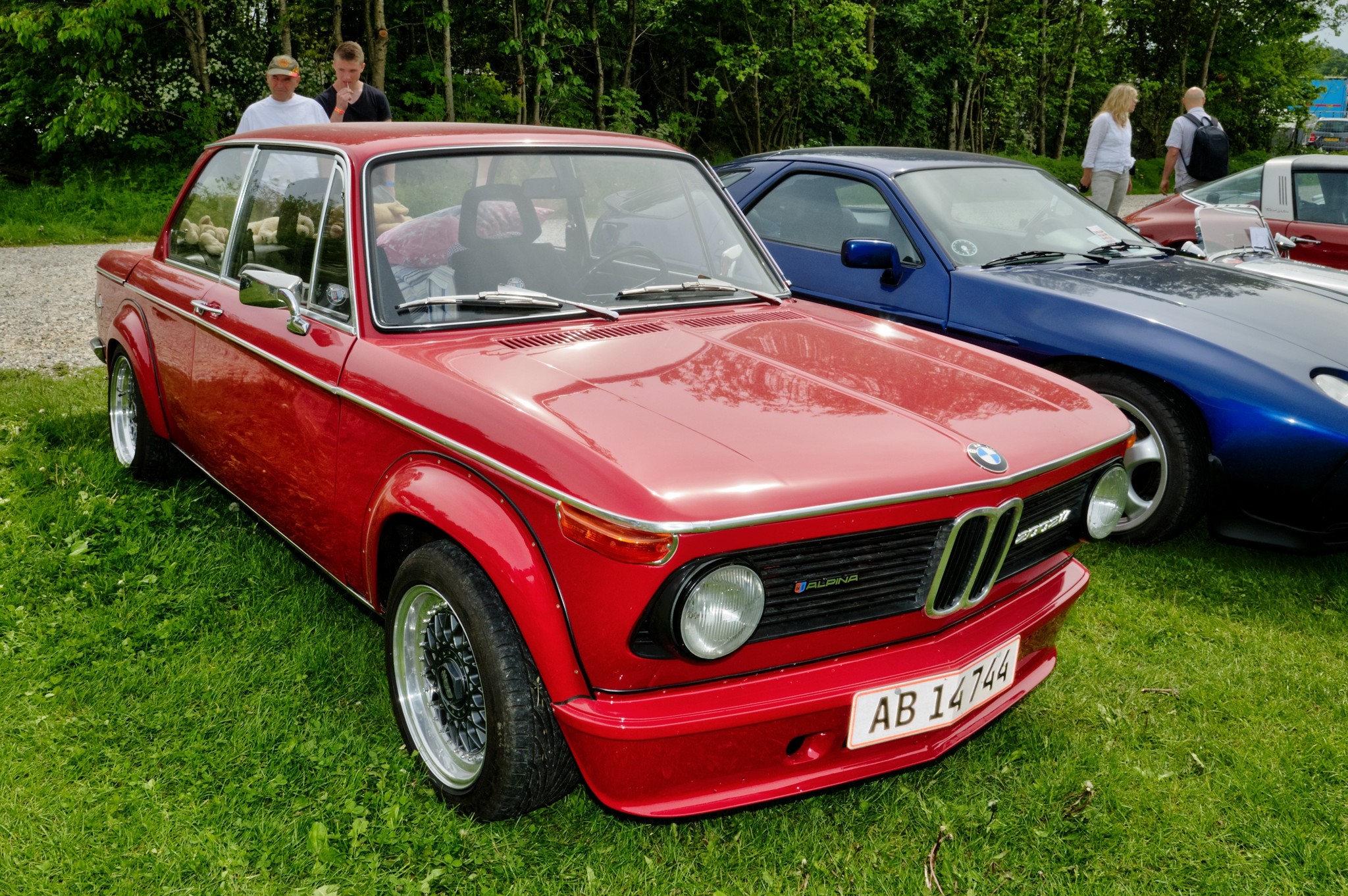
[267,54,300,77]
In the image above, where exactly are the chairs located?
[443,184,577,316]
[886,183,990,268]
[263,174,416,331]
[780,176,845,249]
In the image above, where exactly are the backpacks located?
[1178,112,1229,182]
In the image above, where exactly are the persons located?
[314,40,396,201]
[1159,87,1228,195]
[1079,82,1138,219]
[231,55,335,217]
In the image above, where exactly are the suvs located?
[1307,117,1348,150]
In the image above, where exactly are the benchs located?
[186,243,286,274]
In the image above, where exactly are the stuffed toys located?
[245,213,318,242]
[177,215,230,255]
[328,200,415,239]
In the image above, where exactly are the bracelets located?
[334,106,345,115]
[383,181,394,187]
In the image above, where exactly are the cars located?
[1116,150,1347,271]
[591,145,1345,565]
[82,117,1139,831]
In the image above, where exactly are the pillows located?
[375,199,555,266]
